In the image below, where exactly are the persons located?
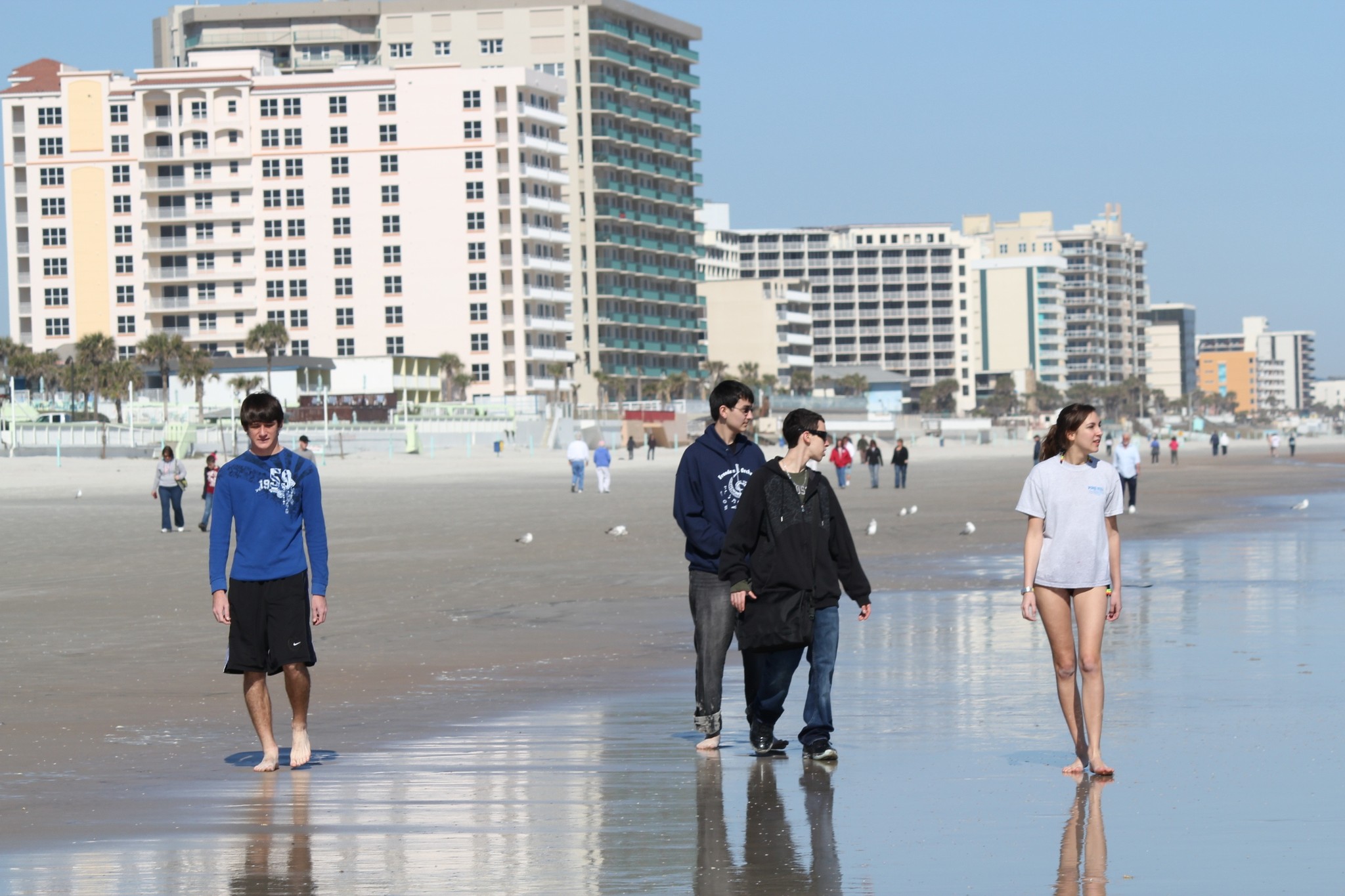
[151,431,1296,535]
[830,440,852,486]
[889,438,910,488]
[594,440,612,495]
[627,435,635,460]
[647,433,656,460]
[1150,436,1160,464]
[721,408,872,761]
[1016,402,1128,776]
[206,389,328,776]
[676,379,767,751]
[1169,436,1179,465]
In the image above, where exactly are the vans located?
[35,412,71,423]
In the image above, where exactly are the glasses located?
[797,428,827,441]
[733,406,753,414]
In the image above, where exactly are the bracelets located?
[1021,587,1035,595]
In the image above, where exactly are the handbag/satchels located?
[734,587,817,652]
[175,459,187,491]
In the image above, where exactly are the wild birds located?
[74,489,83,499]
[864,517,878,536]
[897,504,918,517]
[514,531,534,544]
[1289,498,1309,511]
[958,521,977,537]
[604,525,629,537]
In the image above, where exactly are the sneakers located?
[749,717,773,754]
[803,738,838,761]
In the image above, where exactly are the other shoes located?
[162,528,170,532]
[1129,505,1135,514]
[578,489,582,493]
[199,522,206,532]
[571,483,576,492]
[178,526,184,532]
